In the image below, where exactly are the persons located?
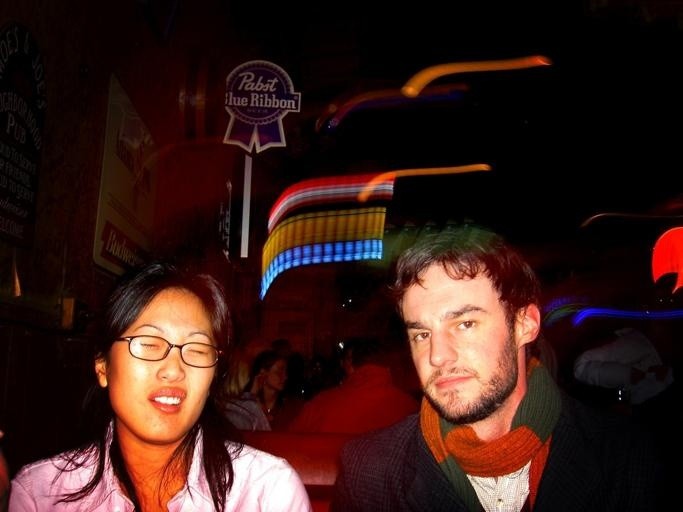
[9,262,311,512]
[331,226,652,512]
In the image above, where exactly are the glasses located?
[116,335,219,368]
